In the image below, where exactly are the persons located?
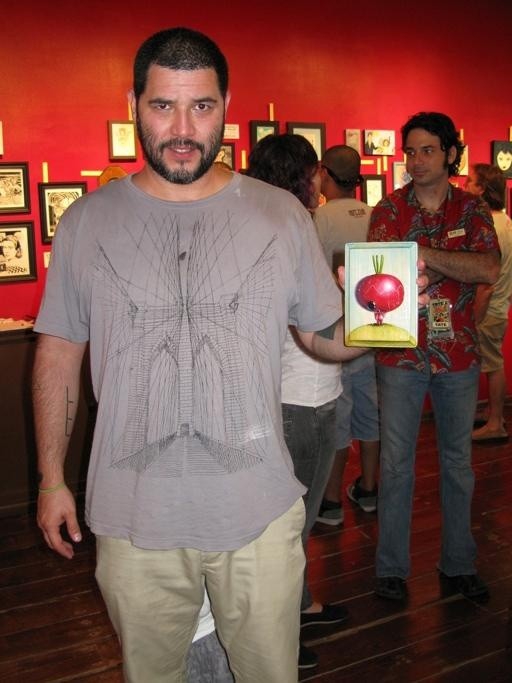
[311,144,378,531]
[245,131,350,673]
[364,132,393,155]
[461,162,512,443]
[495,143,512,171]
[26,25,434,683]
[1,234,26,273]
[368,111,502,607]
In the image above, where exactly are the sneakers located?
[316,494,344,526]
[472,410,508,443]
[438,568,489,597]
[298,642,319,669]
[375,576,408,599]
[345,475,378,513]
[300,603,349,628]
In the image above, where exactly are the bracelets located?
[38,481,65,494]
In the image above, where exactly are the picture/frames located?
[250,120,280,148]
[213,142,235,171]
[107,119,137,160]
[360,174,386,208]
[345,241,419,347]
[393,162,413,190]
[37,182,88,244]
[0,220,37,283]
[287,122,326,161]
[0,161,31,214]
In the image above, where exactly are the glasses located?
[321,165,364,188]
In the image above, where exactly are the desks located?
[0,329,99,519]
